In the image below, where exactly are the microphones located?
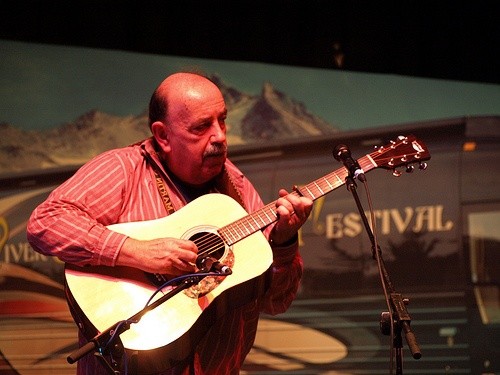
[333,144,367,183]
[196,253,233,275]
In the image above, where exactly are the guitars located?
[64,134,433,375]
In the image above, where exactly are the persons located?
[26,72,314,374]
